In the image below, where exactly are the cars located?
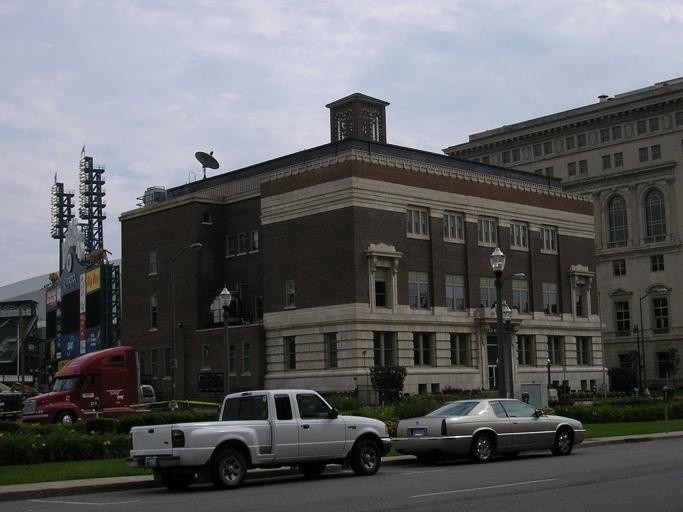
[0,384,30,418]
[396,397,587,462]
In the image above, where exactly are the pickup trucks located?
[130,385,392,485]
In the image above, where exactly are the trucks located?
[20,345,221,425]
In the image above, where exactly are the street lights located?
[639,288,667,398]
[490,245,514,398]
[218,286,234,399]
